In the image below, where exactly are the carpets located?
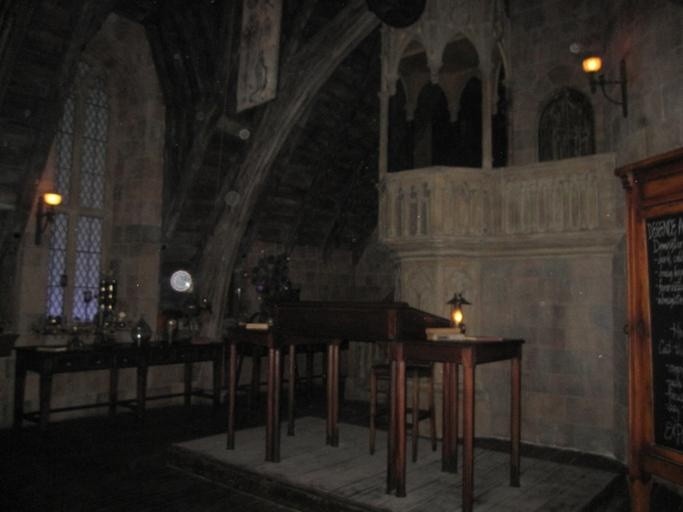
[149,414,627,511]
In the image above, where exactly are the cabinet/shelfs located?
[611,147,683,512]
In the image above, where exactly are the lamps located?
[568,33,629,119]
[35,192,63,246]
